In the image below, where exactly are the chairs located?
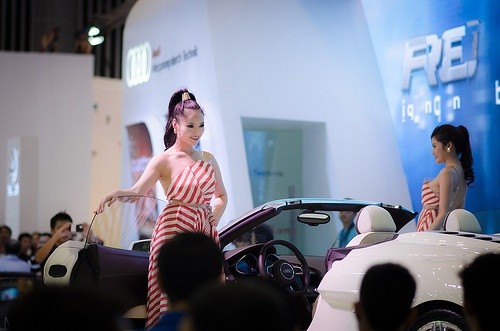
[344,205,396,250]
[444,208,483,232]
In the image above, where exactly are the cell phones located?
[69,224,83,232]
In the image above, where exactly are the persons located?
[416,123,474,232]
[0,211,104,331]
[147,195,499,331]
[94,87,229,329]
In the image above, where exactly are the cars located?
[40,194,500,331]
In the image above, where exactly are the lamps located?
[87,22,106,47]
